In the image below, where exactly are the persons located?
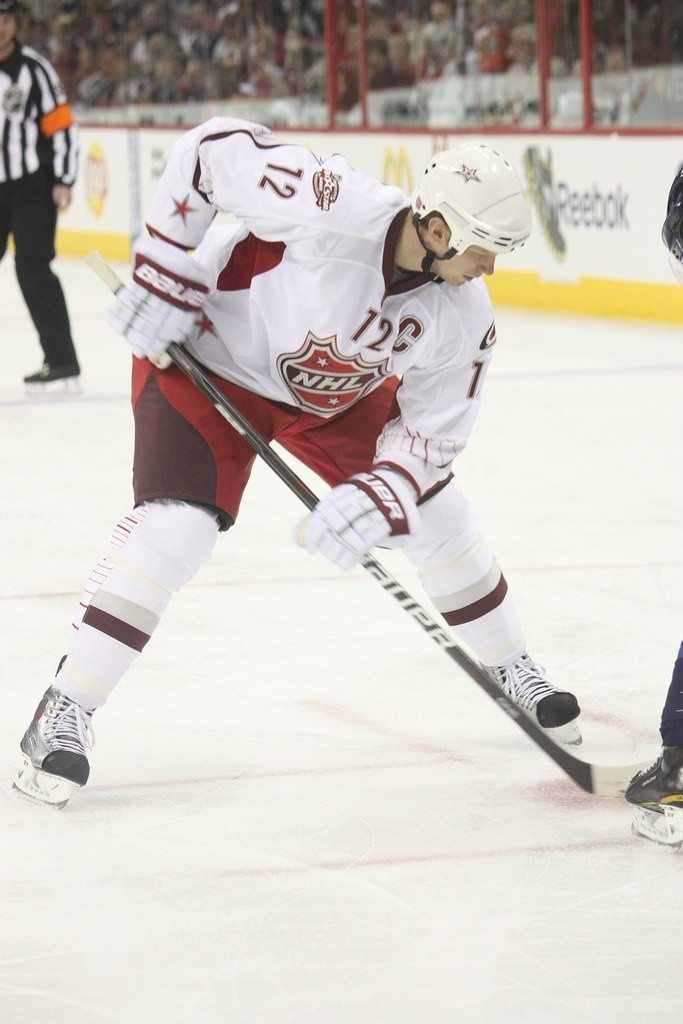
[7,0,683,127]
[12,115,583,808]
[624,157,683,847]
[0,0,82,393]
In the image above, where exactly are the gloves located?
[105,235,214,368]
[294,469,421,570]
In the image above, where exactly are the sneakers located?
[10,685,98,810]
[479,647,583,746]
[623,747,683,853]
[24,360,82,395]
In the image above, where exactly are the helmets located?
[0,0,18,11]
[411,142,534,256]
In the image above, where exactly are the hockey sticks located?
[82,249,672,796]
